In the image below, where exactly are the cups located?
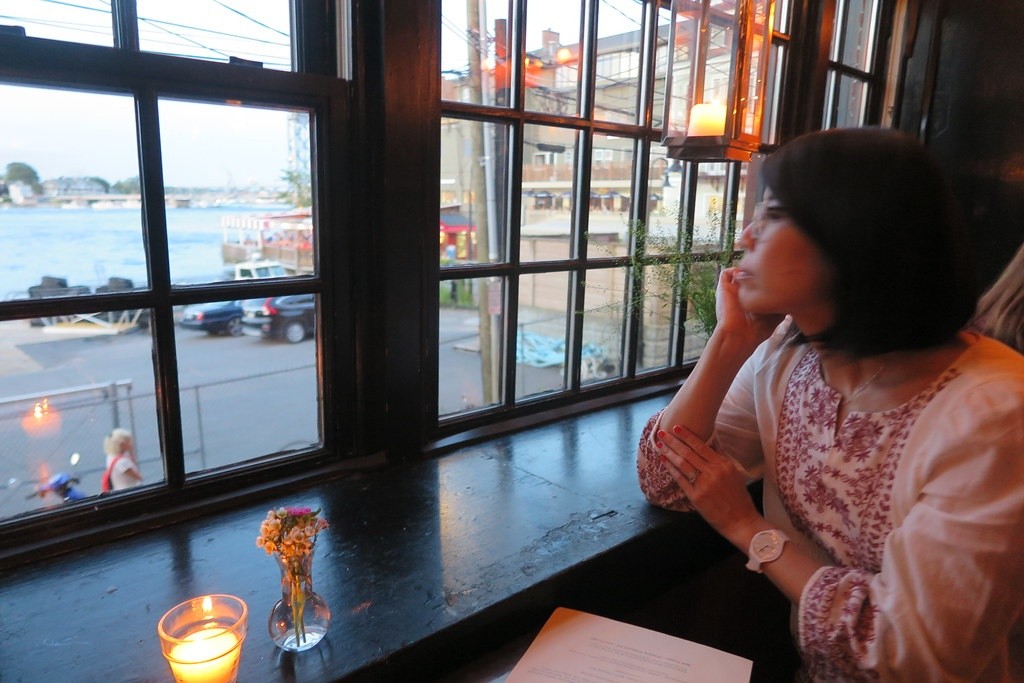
[157,592,248,683]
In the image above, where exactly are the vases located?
[268,545,329,653]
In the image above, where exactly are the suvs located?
[239,292,315,344]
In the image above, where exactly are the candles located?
[686,104,726,136]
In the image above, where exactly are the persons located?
[636,128,1023,683]
[103,428,144,490]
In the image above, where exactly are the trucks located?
[235,261,286,281]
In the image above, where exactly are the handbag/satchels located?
[102,457,121,491]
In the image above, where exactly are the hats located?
[49,472,69,490]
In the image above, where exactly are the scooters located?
[14,451,86,504]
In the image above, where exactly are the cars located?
[179,298,244,339]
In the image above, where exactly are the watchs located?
[744,529,791,571]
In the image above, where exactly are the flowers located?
[255,506,329,647]
